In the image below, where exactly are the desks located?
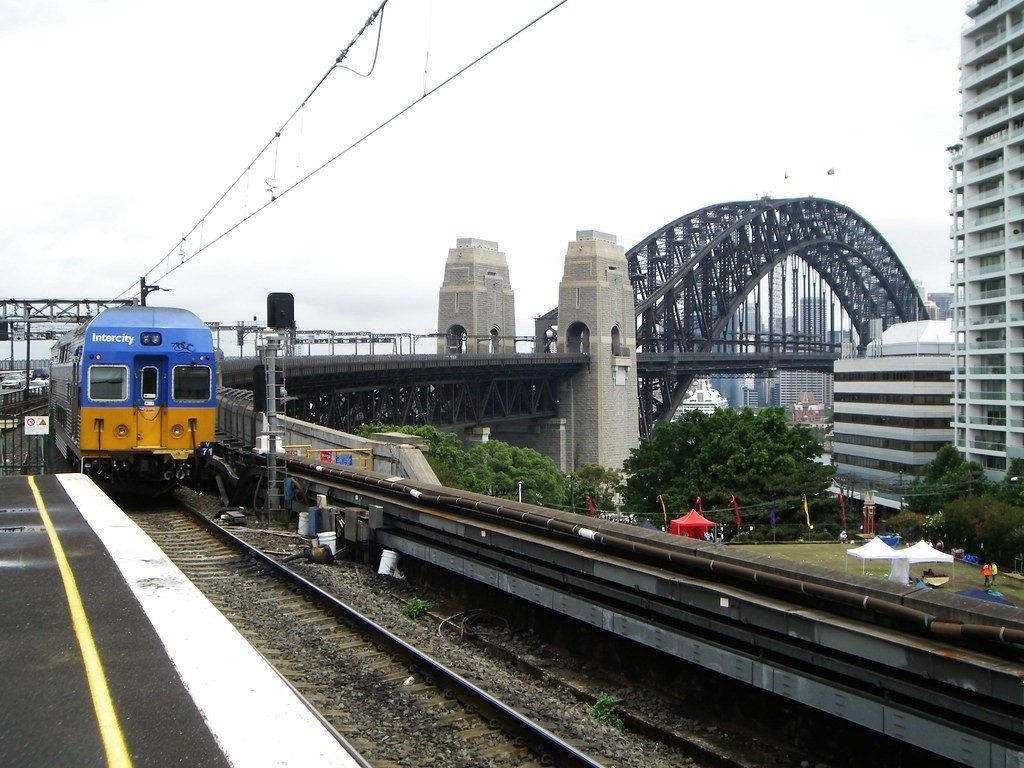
[924,577,949,589]
[856,534,874,544]
[881,537,899,547]
[1003,573,1024,589]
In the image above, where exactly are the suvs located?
[28,367,49,386]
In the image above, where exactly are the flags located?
[586,495,594,516]
[838,492,846,523]
[696,496,703,517]
[658,494,667,521]
[770,502,775,528]
[729,495,741,525]
[802,494,810,526]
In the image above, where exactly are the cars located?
[1,374,26,389]
[21,380,48,396]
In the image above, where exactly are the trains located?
[49,306,216,497]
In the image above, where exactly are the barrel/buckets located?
[318,531,336,555]
[298,512,309,535]
[377,549,399,576]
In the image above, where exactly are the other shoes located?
[987,585,990,587]
[994,585,997,586]
[990,583,993,585]
[984,583,986,585]
[841,542,843,544]
[845,541,847,543]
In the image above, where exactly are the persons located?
[681,528,688,538]
[936,538,944,552]
[716,528,724,544]
[927,568,934,577]
[840,530,847,545]
[704,529,714,542]
[981,561,998,587]
[926,537,933,548]
[599,511,631,526]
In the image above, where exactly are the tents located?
[846,536,955,593]
[671,509,717,543]
